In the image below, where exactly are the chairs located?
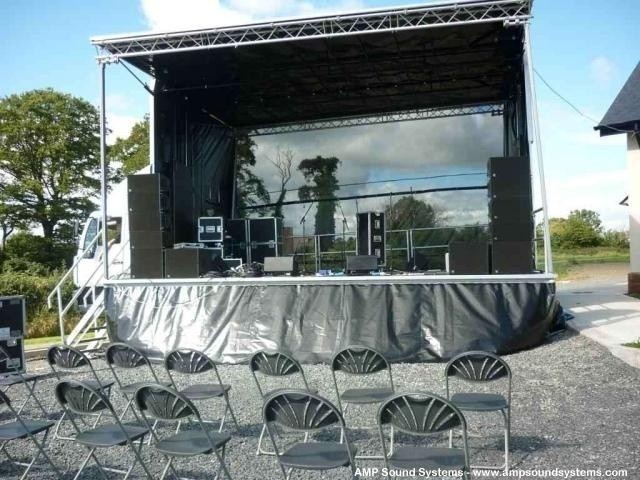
[0,344,511,480]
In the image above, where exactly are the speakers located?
[345,255,379,275]
[164,245,224,278]
[127,173,174,279]
[486,156,535,275]
[449,241,491,275]
[263,256,298,276]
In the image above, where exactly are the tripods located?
[299,194,317,275]
